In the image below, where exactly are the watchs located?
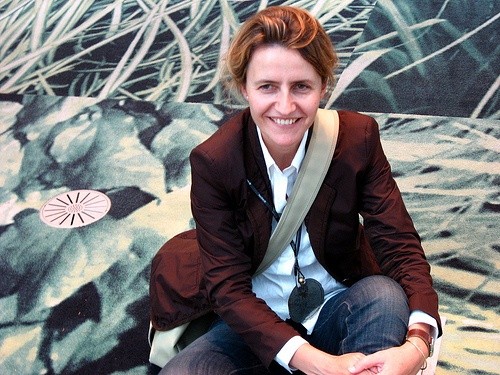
[406,329,434,357]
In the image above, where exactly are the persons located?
[150,5,443,375]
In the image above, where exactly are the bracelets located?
[405,339,428,370]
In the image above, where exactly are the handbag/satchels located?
[148,322,204,368]
